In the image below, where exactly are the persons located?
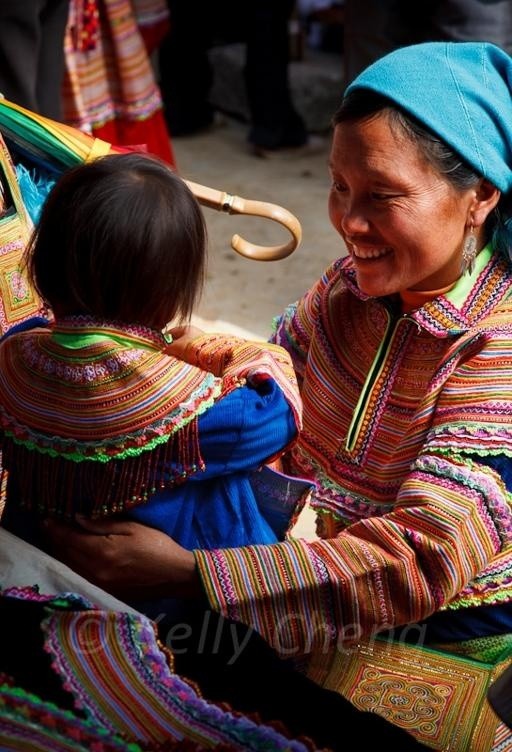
[1,2,511,176]
[0,152,305,614]
[41,38,512,751]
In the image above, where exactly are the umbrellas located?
[1,90,297,265]
